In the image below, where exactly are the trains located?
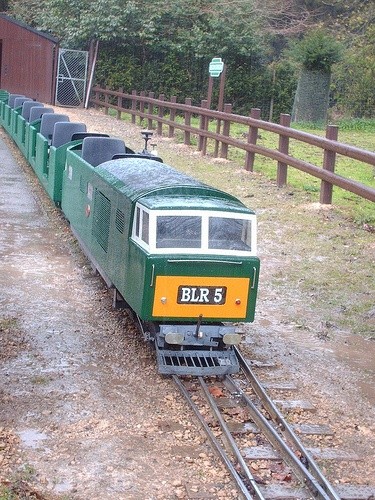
[1,91,259,381]
